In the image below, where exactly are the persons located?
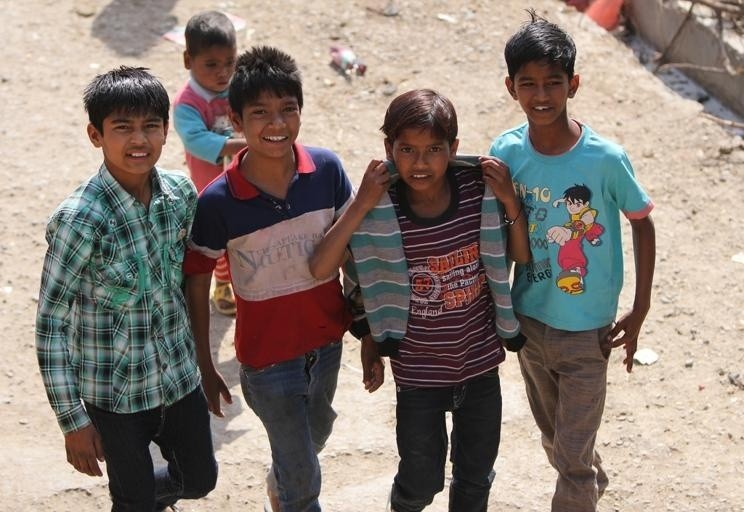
[181,46,387,511]
[33,65,220,511]
[174,11,250,318]
[489,10,660,512]
[307,85,533,511]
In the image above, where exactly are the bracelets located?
[500,202,526,226]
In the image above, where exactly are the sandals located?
[212,283,238,314]
